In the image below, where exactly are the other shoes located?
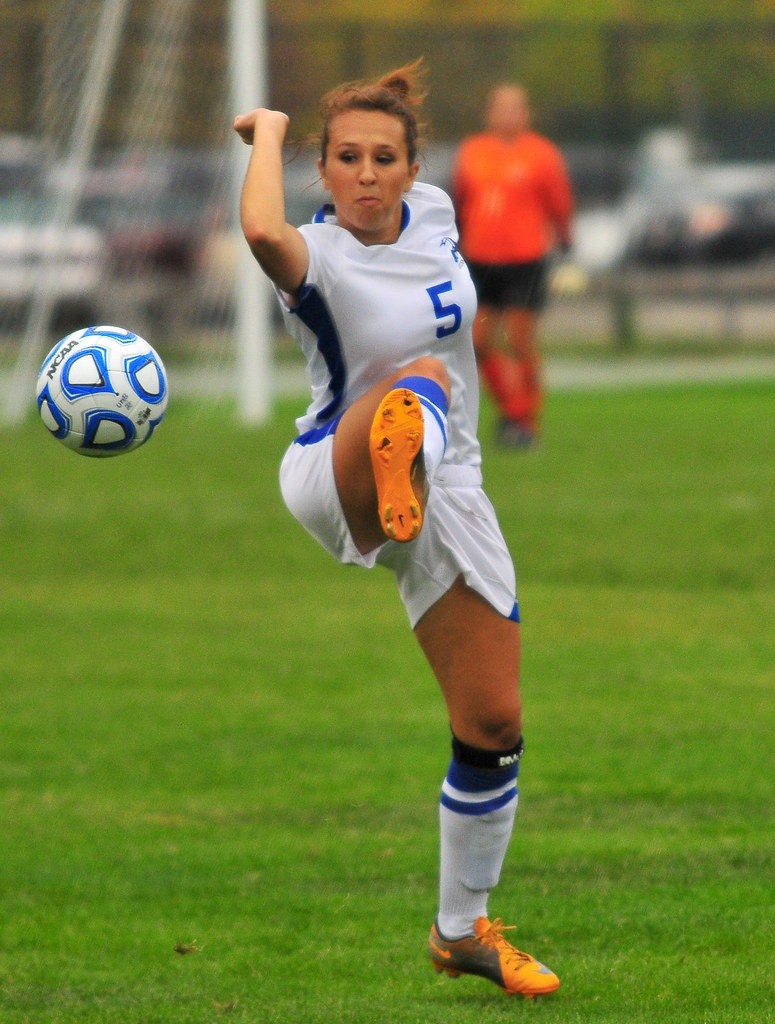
[495,415,536,454]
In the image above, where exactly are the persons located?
[449,82,574,447]
[232,57,560,997]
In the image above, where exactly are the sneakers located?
[429,916,560,1001]
[369,388,429,544]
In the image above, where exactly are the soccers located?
[35,324,170,459]
